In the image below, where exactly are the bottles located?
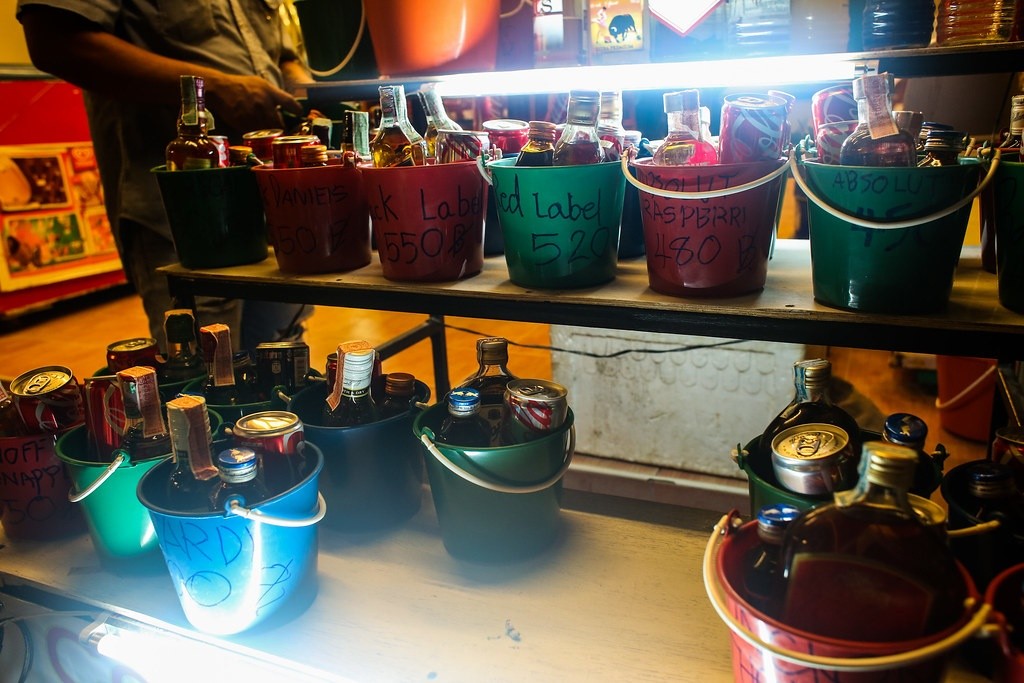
[746,359,937,641]
[437,336,517,448]
[530,1,650,70]
[162,309,255,407]
[320,342,418,427]
[166,72,1024,168]
[166,395,274,511]
[1,384,27,437]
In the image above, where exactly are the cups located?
[726,1,790,60]
[935,0,1023,47]
[847,0,937,53]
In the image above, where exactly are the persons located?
[14,0,327,354]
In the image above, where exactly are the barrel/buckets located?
[138,422,327,641]
[930,461,1024,683]
[733,428,950,514]
[153,143,1023,316]
[180,367,317,441]
[288,372,431,530]
[362,0,499,79]
[702,510,990,683]
[0,355,223,573]
[413,401,574,563]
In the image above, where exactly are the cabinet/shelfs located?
[0,62,1024,683]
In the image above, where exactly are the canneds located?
[769,422,856,500]
[255,341,310,393]
[8,336,160,462]
[326,353,338,396]
[716,84,859,166]
[989,425,1024,506]
[206,128,321,169]
[500,378,568,445]
[906,492,949,549]
[232,410,306,498]
[433,119,529,164]
